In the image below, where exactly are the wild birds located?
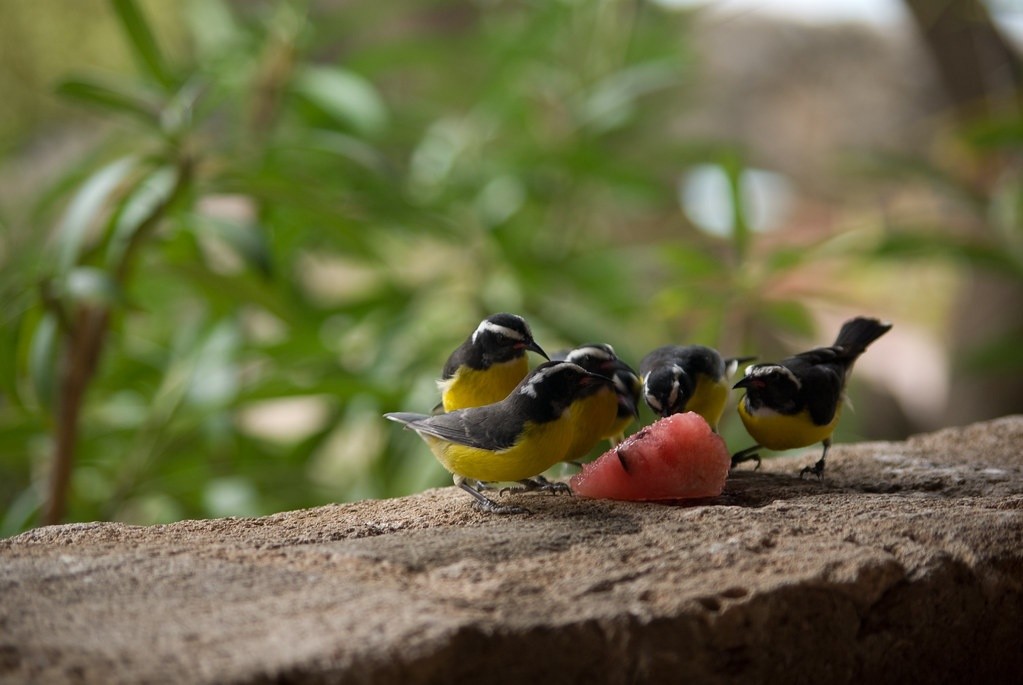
[382,310,760,516]
[729,315,893,482]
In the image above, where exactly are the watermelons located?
[569,412,731,500]
